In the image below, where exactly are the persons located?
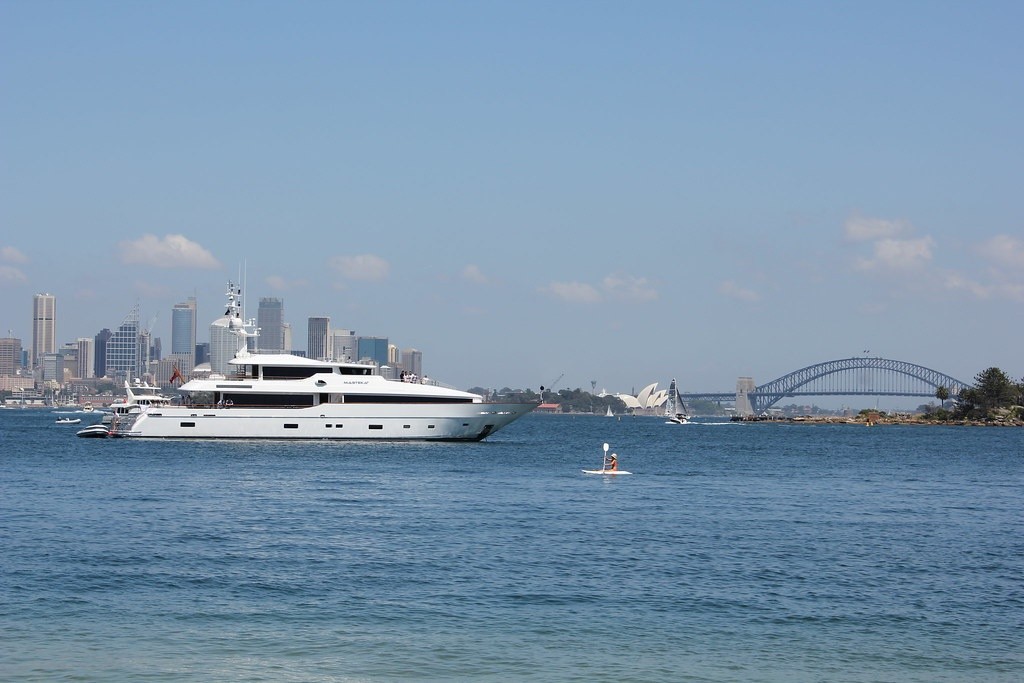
[400,371,418,384]
[604,453,617,472]
[186,395,191,404]
[217,400,224,409]
[179,396,185,405]
[225,398,233,409]
[422,375,430,385]
[111,410,119,430]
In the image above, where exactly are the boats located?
[113,280,548,444]
[105,382,172,423]
[580,467,632,475]
[77,424,110,439]
[54,416,83,426]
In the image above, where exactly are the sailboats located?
[662,376,701,425]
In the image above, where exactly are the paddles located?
[601,443,609,473]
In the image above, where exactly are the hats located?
[611,454,617,459]
